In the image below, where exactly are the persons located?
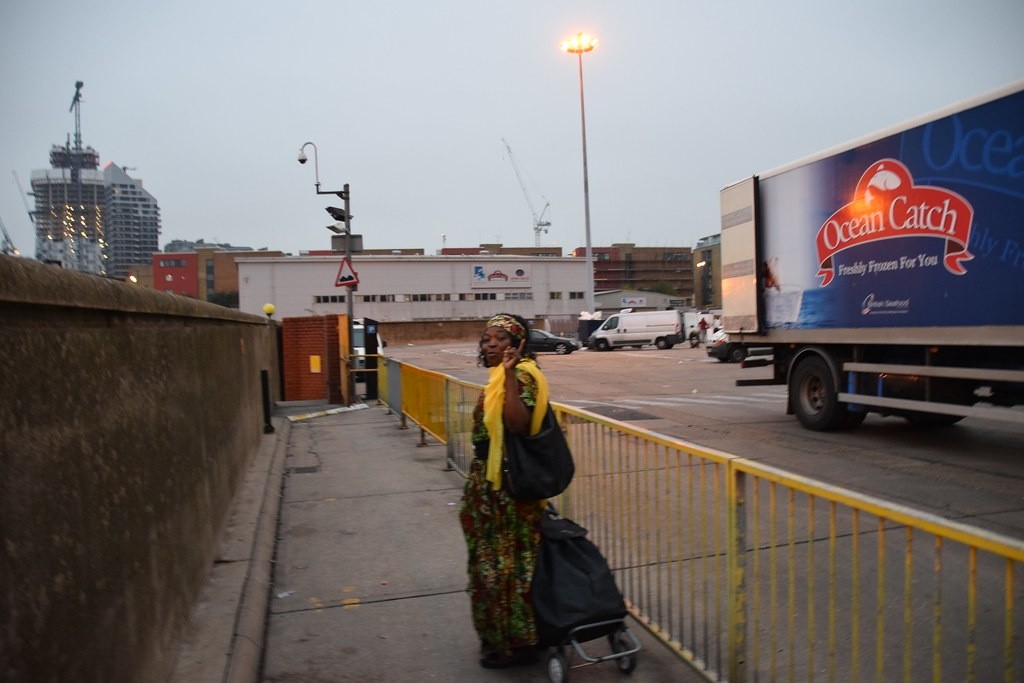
[714,317,721,331]
[699,317,708,343]
[460,311,556,666]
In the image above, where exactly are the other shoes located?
[481,648,538,670]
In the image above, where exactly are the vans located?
[706,328,776,363]
[350,321,389,385]
[587,310,685,352]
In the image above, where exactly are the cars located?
[526,328,582,356]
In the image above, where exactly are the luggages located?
[527,501,643,682]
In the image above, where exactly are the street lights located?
[298,139,357,402]
[560,32,603,314]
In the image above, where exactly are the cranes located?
[67,77,86,271]
[502,136,554,248]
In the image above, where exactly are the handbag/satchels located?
[503,401,575,503]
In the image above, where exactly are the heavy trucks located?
[718,76,1024,432]
